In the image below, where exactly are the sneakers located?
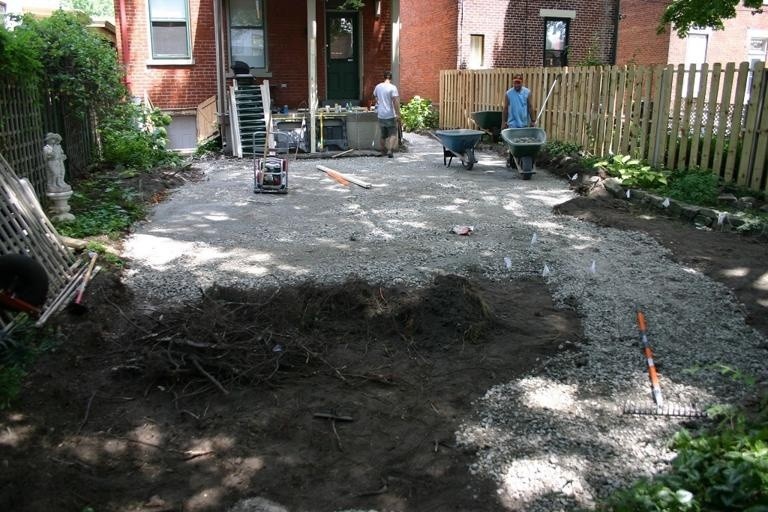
[387,151,392,158]
[381,147,388,155]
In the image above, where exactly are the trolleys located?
[252,130,293,195]
[425,127,485,170]
[500,125,548,178]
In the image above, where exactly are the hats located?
[512,75,522,82]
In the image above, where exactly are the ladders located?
[229,80,276,159]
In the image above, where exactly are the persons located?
[42,132,64,193]
[54,134,71,191]
[373,70,403,158]
[500,73,536,168]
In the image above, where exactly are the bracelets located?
[531,120,535,122]
